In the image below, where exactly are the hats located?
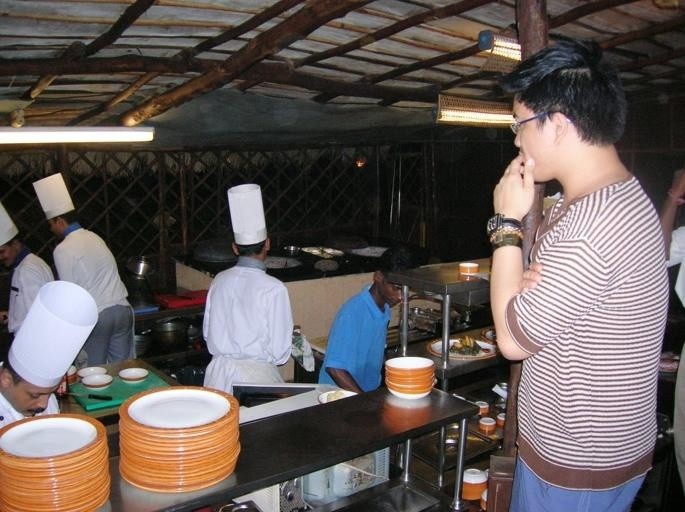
[0,200,18,248]
[32,172,75,220]
[7,280,99,389]
[228,183,267,245]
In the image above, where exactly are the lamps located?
[433,93,515,127]
[477,27,520,60]
[1,124,158,145]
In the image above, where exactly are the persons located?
[0,234,55,345]
[484,40,670,512]
[317,245,428,394]
[33,208,136,365]
[0,357,98,426]
[202,238,295,400]
[660,169,684,496]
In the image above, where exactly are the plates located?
[425,338,498,359]
[385,356,439,400]
[319,389,359,404]
[481,326,498,344]
[67,365,149,391]
[461,467,488,511]
[0,385,242,512]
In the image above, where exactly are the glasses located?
[510,112,573,134]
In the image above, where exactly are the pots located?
[134,319,202,356]
[124,255,155,291]
[189,241,239,277]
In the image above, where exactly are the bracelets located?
[489,228,523,248]
[666,191,683,206]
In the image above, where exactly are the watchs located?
[486,213,523,234]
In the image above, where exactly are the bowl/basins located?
[283,246,301,256]
[475,400,506,435]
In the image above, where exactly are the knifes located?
[67,392,113,399]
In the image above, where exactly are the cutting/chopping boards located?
[69,370,171,411]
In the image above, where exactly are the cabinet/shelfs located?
[401,257,510,495]
[96,384,480,512]
[118,251,211,367]
